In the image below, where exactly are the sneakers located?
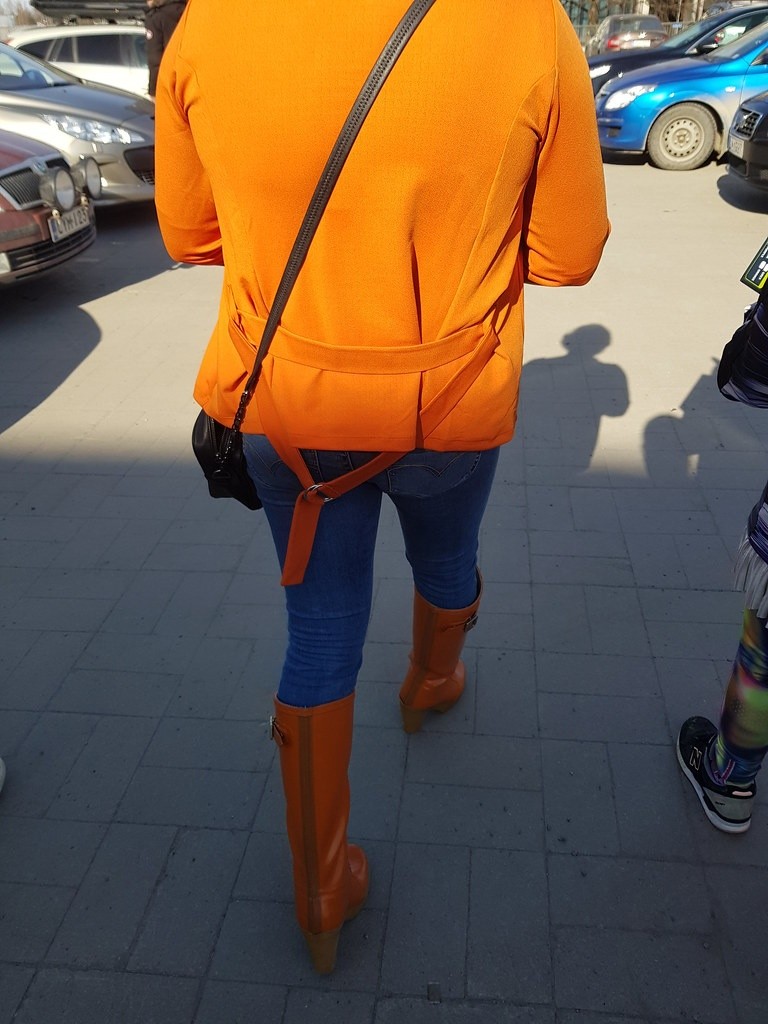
[677,716,757,833]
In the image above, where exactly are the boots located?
[269,693,368,977]
[399,566,484,733]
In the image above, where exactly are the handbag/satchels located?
[191,409,262,510]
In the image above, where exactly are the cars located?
[1,1,179,285]
[579,1,767,188]
